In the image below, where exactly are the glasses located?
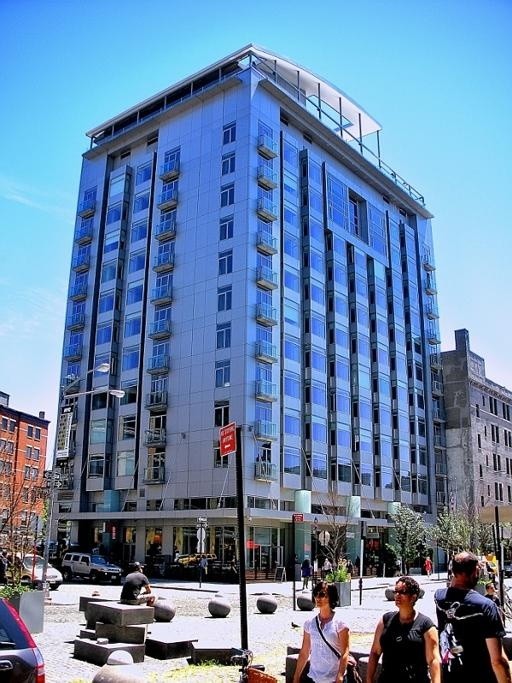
[392,588,407,595]
[313,591,328,597]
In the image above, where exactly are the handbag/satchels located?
[346,663,363,683]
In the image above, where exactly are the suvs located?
[61,552,122,585]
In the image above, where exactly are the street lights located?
[39,362,126,594]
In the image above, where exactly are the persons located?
[424,556,432,578]
[433,551,512,683]
[292,580,350,683]
[367,576,442,683]
[300,558,312,589]
[484,583,501,609]
[322,557,354,579]
[394,558,403,577]
[201,555,210,580]
[120,564,156,606]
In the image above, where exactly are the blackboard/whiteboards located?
[274,567,285,579]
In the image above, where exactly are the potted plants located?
[0,581,44,635]
[324,566,352,606]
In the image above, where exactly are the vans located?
[0,552,63,591]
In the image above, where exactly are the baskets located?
[246,667,278,683]
